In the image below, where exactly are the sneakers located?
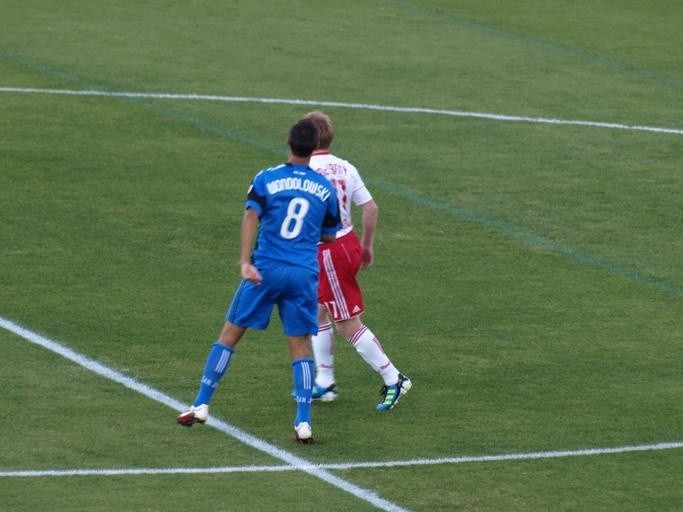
[294,421,315,444]
[377,373,411,409]
[176,403,208,427]
[293,381,338,402]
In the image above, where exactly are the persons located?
[291,109,412,415]
[175,119,343,440]
